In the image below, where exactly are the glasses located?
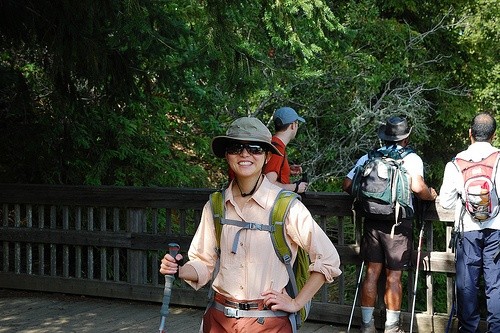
[224,141,269,155]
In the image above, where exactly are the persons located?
[264,107,308,193]
[342,117,437,333]
[160,117,342,333]
[435,113,500,333]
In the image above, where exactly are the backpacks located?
[350,148,415,225]
[209,190,312,333]
[452,150,500,224]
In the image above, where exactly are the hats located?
[213,118,284,157]
[377,117,414,142]
[274,107,305,125]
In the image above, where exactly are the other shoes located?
[384,321,410,333]
[361,319,383,333]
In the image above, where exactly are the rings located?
[305,187,306,190]
[275,295,277,299]
